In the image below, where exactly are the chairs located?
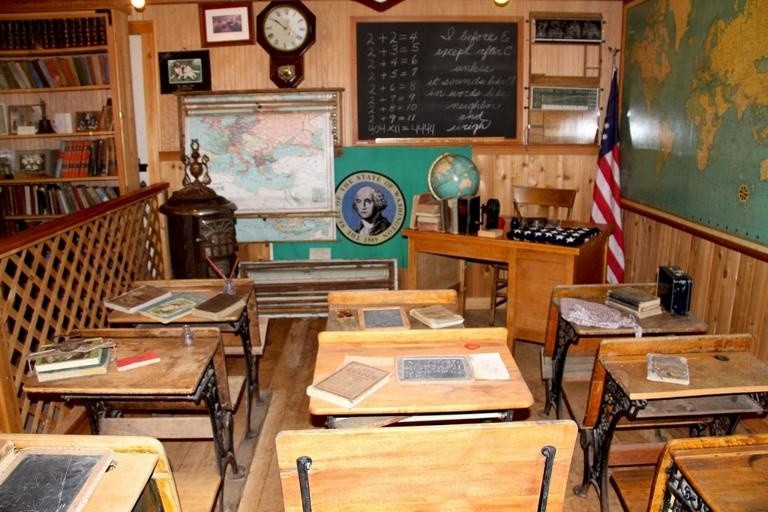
[491,187,576,325]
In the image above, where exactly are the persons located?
[351,185,391,237]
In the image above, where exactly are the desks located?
[273,287,578,511]
[1,277,268,510]
[539,283,768,512]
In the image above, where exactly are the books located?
[409,192,480,235]
[645,353,689,386]
[102,282,246,325]
[605,286,663,319]
[32,338,112,383]
[306,361,393,408]
[409,304,465,330]
[0,15,119,234]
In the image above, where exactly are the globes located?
[428,153,480,234]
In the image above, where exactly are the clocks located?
[256,0,317,89]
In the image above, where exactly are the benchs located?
[239,259,395,313]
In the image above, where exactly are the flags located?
[588,65,626,286]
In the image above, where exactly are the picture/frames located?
[158,50,212,94]
[9,103,44,134]
[75,110,103,133]
[198,0,255,47]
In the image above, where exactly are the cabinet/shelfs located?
[402,216,612,359]
[0,0,140,220]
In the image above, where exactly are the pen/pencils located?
[204,255,228,281]
[229,252,240,279]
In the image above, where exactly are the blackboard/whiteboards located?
[349,16,525,145]
